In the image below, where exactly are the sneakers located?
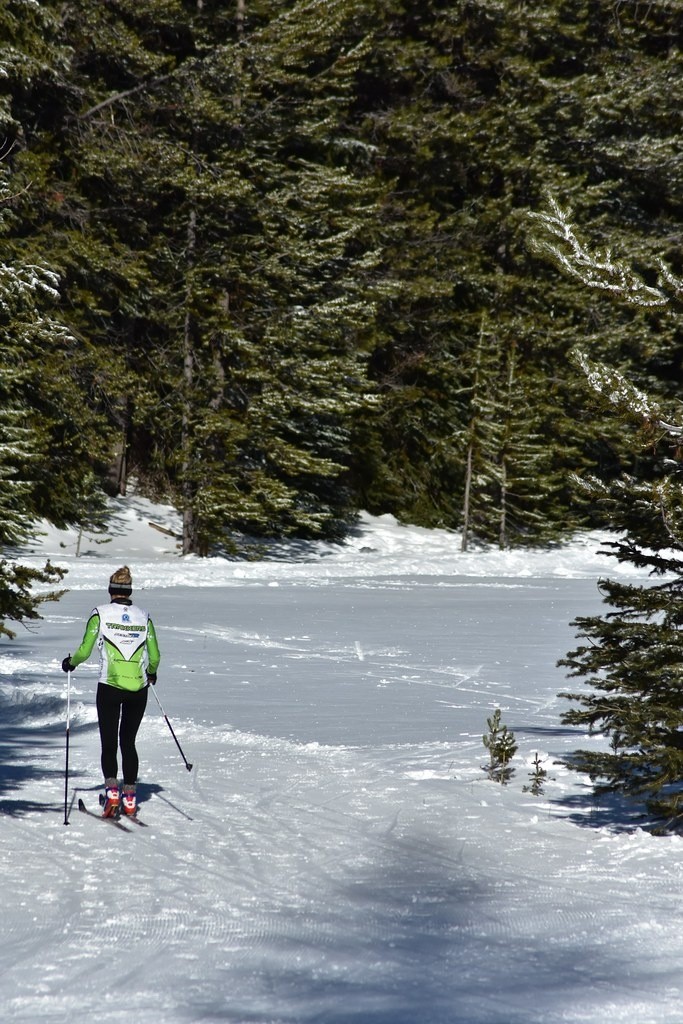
[100,778,120,817]
[121,784,137,817]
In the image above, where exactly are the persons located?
[60,565,161,820]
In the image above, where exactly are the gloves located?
[62,657,75,672]
[145,669,157,685]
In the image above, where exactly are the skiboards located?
[76,797,153,836]
